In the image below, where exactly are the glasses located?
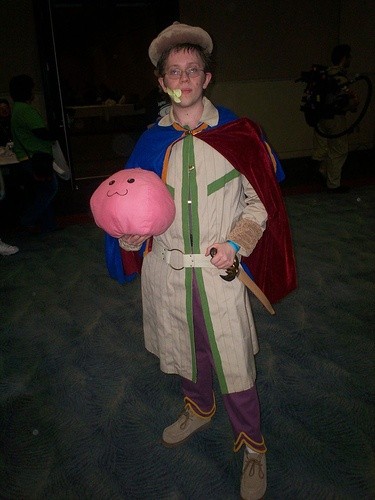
[166,67,205,80]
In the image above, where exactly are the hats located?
[149,21,213,66]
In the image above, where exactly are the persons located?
[0,173,20,256]
[96,20,281,499]
[9,73,67,234]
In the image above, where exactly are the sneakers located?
[240,449,267,500]
[0,241,19,255]
[161,408,216,448]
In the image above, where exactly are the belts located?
[152,238,217,270]
